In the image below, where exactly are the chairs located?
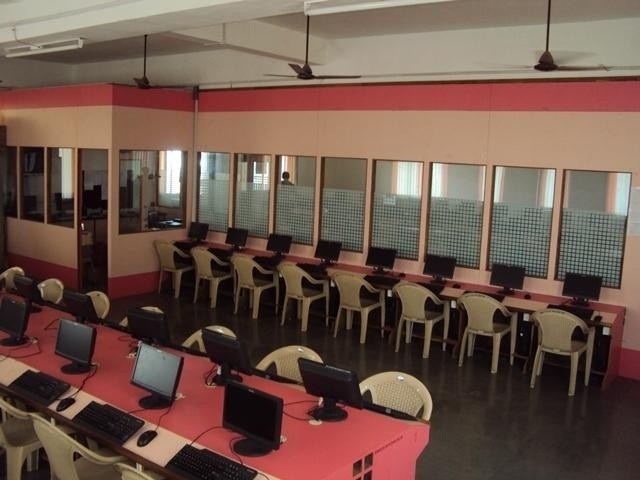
[190,246,236,308]
[153,238,195,299]
[277,262,329,332]
[229,254,279,320]
[330,270,385,344]
[456,291,517,374]
[0,266,433,480]
[391,281,451,359]
[528,308,596,397]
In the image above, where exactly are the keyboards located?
[169,342,209,357]
[71,401,145,445]
[102,319,127,333]
[7,369,71,408]
[254,369,298,384]
[363,403,418,421]
[165,444,258,480]
[547,304,593,321]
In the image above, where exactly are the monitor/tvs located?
[489,262,526,296]
[0,297,31,346]
[222,378,284,458]
[266,233,292,259]
[200,327,255,386]
[561,271,602,304]
[14,195,38,212]
[63,288,101,326]
[314,238,342,268]
[225,228,248,250]
[54,192,73,217]
[366,246,397,276]
[127,307,170,345]
[189,222,209,245]
[422,254,457,287]
[298,357,363,422]
[55,316,97,374]
[13,277,42,313]
[129,340,184,409]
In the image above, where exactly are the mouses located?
[137,430,158,446]
[56,398,75,411]
[593,316,603,326]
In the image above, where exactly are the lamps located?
[5,37,86,58]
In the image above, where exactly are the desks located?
[1,289,431,480]
[172,238,627,391]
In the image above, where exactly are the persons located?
[281,172,294,185]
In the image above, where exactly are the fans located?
[262,15,362,80]
[107,34,187,89]
[495,0,615,72]
